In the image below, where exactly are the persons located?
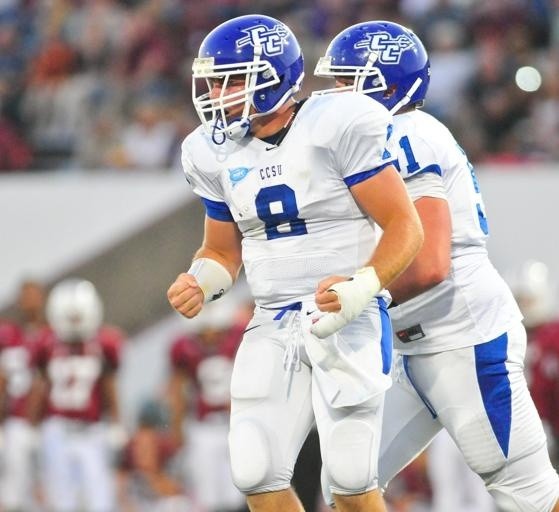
[1,279,252,510]
[168,14,426,512]
[312,20,559,512]
[1,2,559,170]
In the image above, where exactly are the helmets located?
[45,278,105,343]
[314,21,430,110]
[198,14,305,115]
